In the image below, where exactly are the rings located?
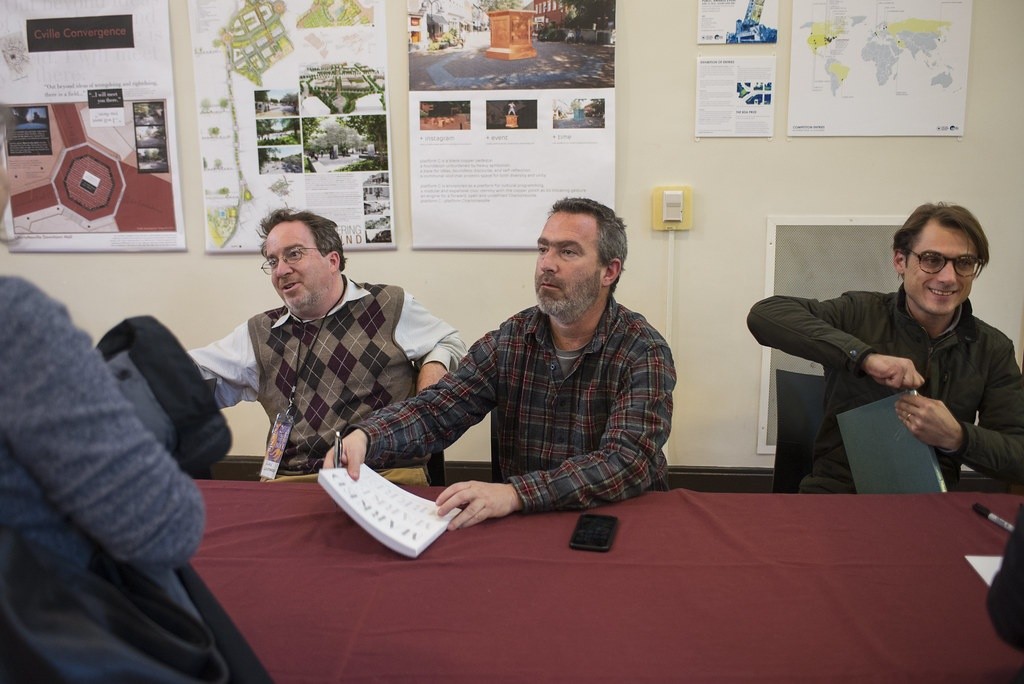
[904,414,911,420]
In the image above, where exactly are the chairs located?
[772,368,828,493]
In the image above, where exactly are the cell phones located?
[570,514,618,552]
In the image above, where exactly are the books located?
[317,463,462,559]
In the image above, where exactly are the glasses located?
[902,247,985,278]
[260,248,341,275]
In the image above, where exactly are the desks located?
[180,480,1024,684]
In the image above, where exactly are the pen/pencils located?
[333,432,342,469]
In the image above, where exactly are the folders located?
[837,389,949,495]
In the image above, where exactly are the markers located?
[973,502,1015,532]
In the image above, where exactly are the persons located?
[747,201,1024,495]
[321,197,677,531]
[0,275,276,684]
[186,207,469,487]
[986,502,1024,652]
